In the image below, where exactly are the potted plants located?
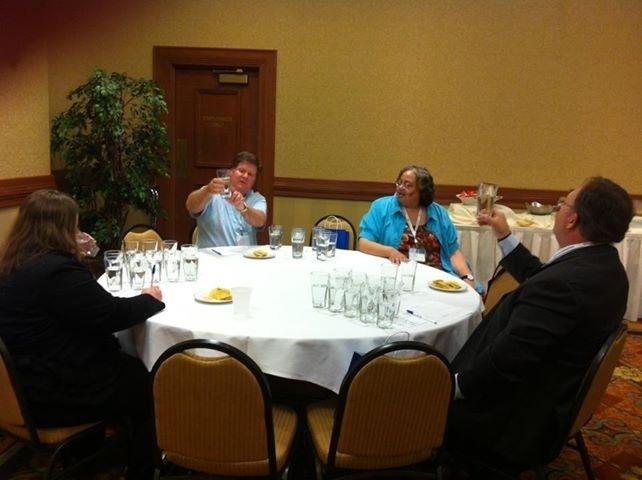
[48,66,176,279]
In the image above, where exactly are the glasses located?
[395,181,418,188]
[557,199,575,212]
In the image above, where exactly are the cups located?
[103,236,202,293]
[289,227,308,261]
[267,223,286,253]
[310,259,418,331]
[475,192,495,229]
[310,224,339,262]
[216,167,233,199]
[73,227,102,259]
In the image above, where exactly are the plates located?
[194,286,243,304]
[426,276,467,292]
[243,247,277,260]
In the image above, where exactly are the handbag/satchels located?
[314,216,349,250]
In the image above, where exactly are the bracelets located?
[240,201,249,214]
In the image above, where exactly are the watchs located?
[460,272,475,282]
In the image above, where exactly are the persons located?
[448,175,634,465]
[357,165,487,304]
[185,150,269,249]
[1,187,166,480]
